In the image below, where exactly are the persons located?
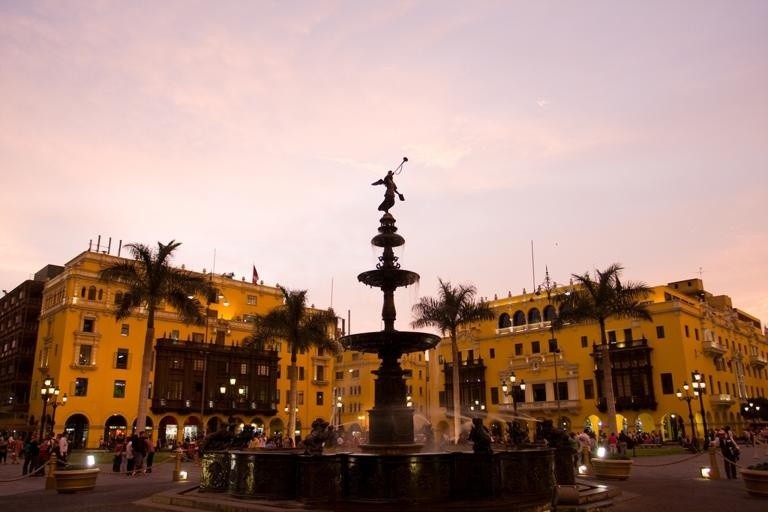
[373,170,405,213]
[704,426,766,446]
[677,429,700,453]
[5,429,69,476]
[467,416,571,477]
[302,417,335,475]
[249,432,369,447]
[199,426,258,453]
[100,430,168,476]
[580,426,661,454]
[711,430,740,478]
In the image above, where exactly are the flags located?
[251,266,260,285]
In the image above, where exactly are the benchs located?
[637,443,662,449]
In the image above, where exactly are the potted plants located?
[740,461,768,496]
[591,454,634,480]
[53,465,100,494]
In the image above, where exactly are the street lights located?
[336,395,343,431]
[674,381,699,450]
[668,412,677,441]
[45,385,67,439]
[691,370,708,444]
[187,268,229,441]
[502,371,526,417]
[528,264,571,427]
[38,374,55,444]
[405,393,413,407]
[218,375,244,434]
[283,402,299,415]
[743,402,760,459]
[469,399,485,420]
[7,392,17,440]
[357,410,365,429]
